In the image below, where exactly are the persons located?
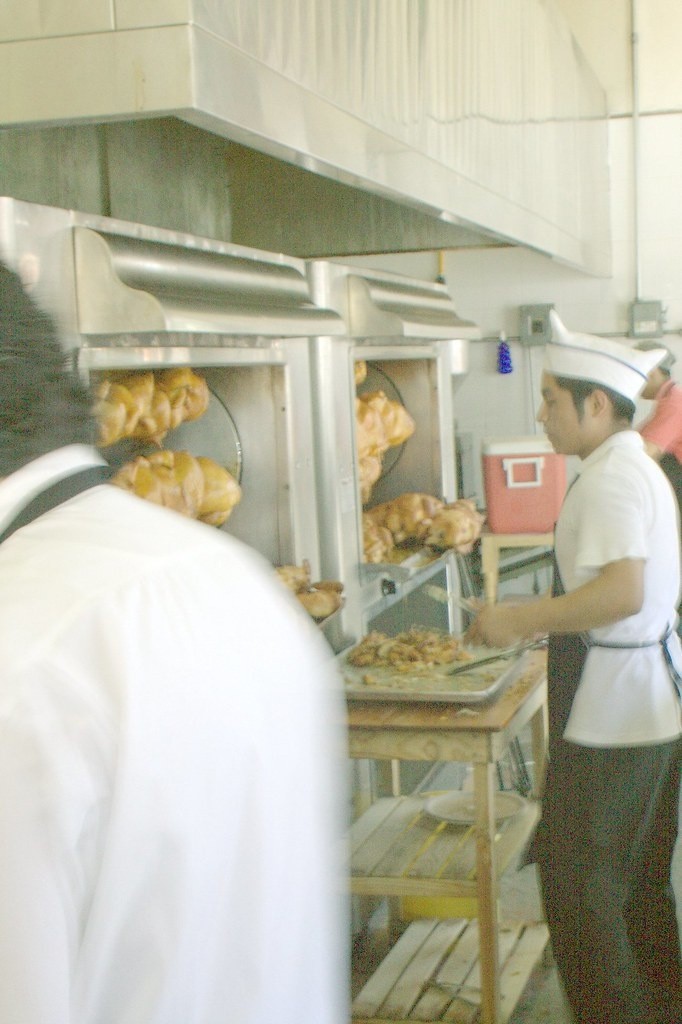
[464,307,681,1023]
[0,258,353,1024]
[634,340,682,505]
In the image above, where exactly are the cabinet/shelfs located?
[345,648,548,1024]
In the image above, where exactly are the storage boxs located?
[481,443,565,536]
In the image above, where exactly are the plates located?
[422,788,528,826]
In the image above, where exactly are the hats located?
[541,312,668,404]
[631,340,676,374]
